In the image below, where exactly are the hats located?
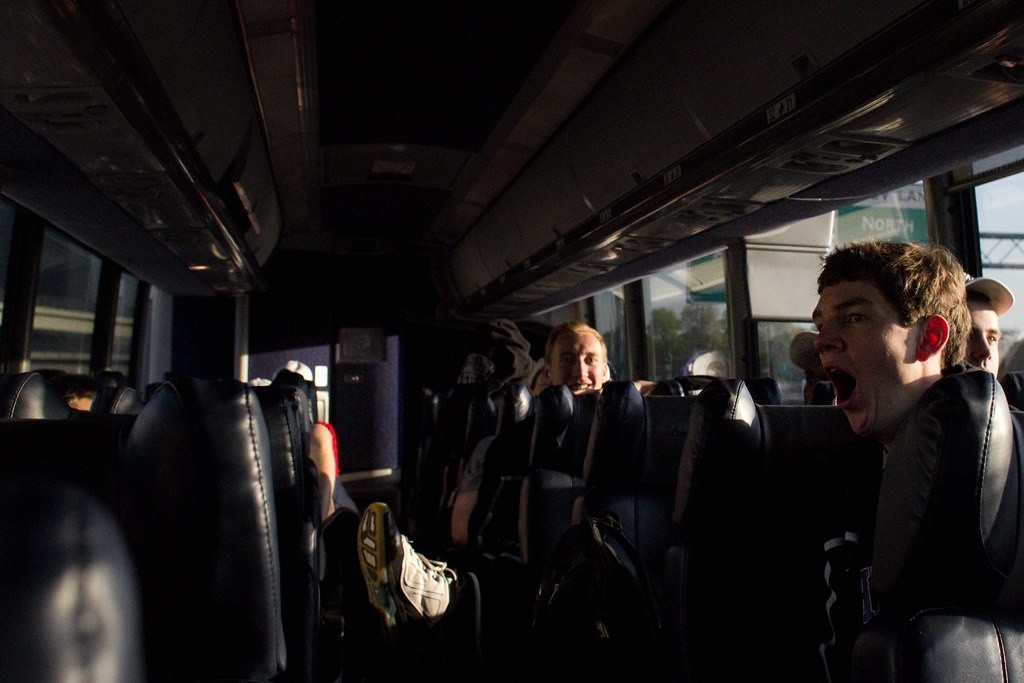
[790,332,824,371]
[250,376,272,386]
[286,358,314,382]
[963,271,1009,311]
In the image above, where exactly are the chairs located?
[0,364,331,683]
[401,381,864,683]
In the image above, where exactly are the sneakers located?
[358,502,457,648]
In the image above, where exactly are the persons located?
[269,239,1024,683]
